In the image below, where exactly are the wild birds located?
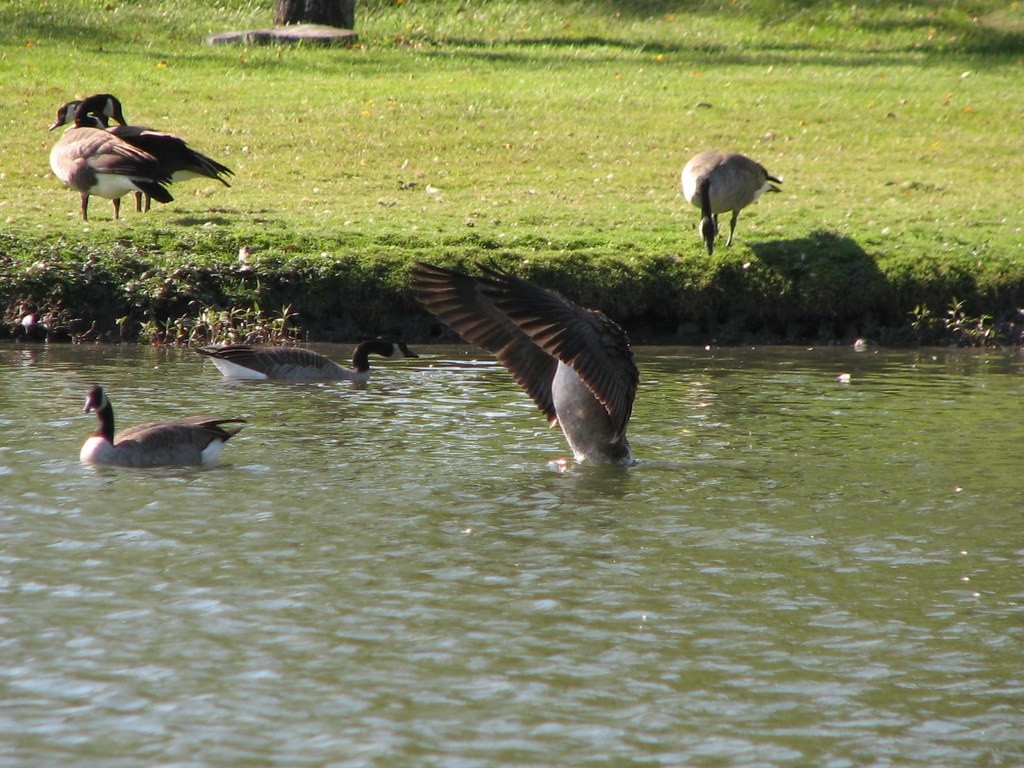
[196,333,419,383]
[681,150,783,256]
[80,385,249,468]
[48,94,173,224]
[411,260,643,468]
[48,98,235,215]
